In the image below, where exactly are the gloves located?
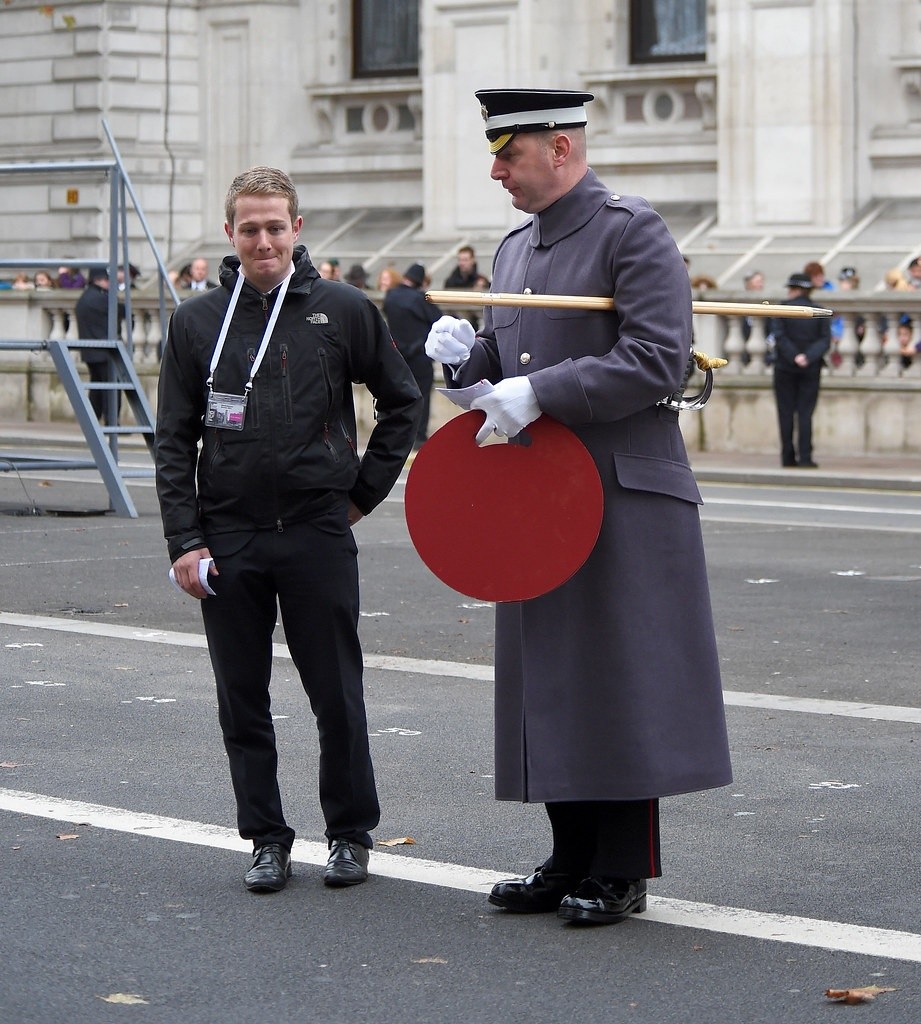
[424,315,476,366]
[469,375,543,445]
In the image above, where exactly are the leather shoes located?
[323,839,370,886]
[488,866,577,910]
[557,873,647,923]
[244,845,292,891]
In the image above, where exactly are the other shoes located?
[782,448,818,466]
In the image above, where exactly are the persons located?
[152,166,427,891]
[691,256,921,374]
[769,273,832,470]
[74,268,134,437]
[420,87,734,928]
[11,246,492,364]
[379,264,442,451]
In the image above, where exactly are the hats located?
[89,266,110,279]
[836,266,856,280]
[787,275,815,288]
[472,88,596,156]
[398,264,425,290]
[341,262,371,285]
[118,263,140,278]
